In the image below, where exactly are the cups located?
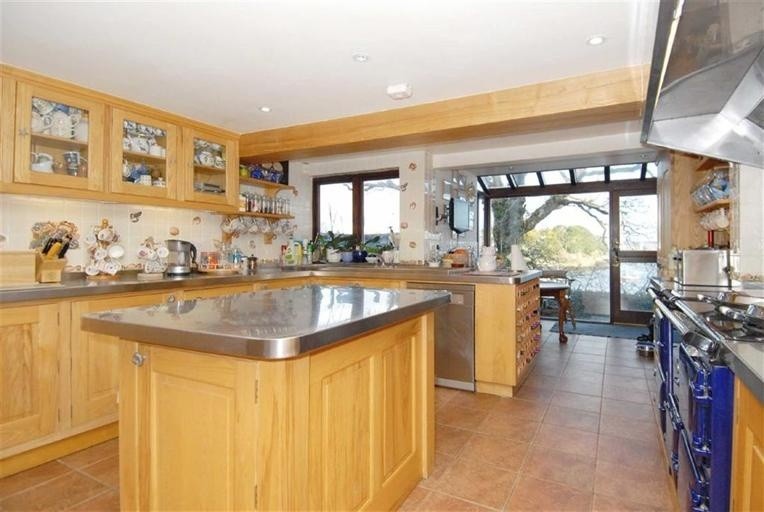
[194,138,226,171]
[122,159,166,189]
[219,214,298,236]
[85,228,124,277]
[700,209,730,233]
[137,240,170,274]
[30,150,87,178]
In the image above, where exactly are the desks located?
[540,283,570,342]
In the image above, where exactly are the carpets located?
[549,319,649,340]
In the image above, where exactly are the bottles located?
[239,192,291,215]
[201,241,258,277]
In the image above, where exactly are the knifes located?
[40,234,72,259]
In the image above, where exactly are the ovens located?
[671,248,733,287]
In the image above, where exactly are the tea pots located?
[31,96,89,146]
[123,119,167,158]
[476,250,498,274]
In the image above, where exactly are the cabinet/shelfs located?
[109,97,241,211]
[163,277,303,304]
[238,176,299,220]
[651,304,763,510]
[119,340,304,512]
[694,153,730,211]
[60,290,163,459]
[3,298,58,478]
[12,81,107,191]
[475,278,541,400]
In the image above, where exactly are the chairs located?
[541,270,577,327]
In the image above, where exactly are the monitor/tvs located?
[452,198,469,231]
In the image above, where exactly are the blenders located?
[165,239,199,275]
